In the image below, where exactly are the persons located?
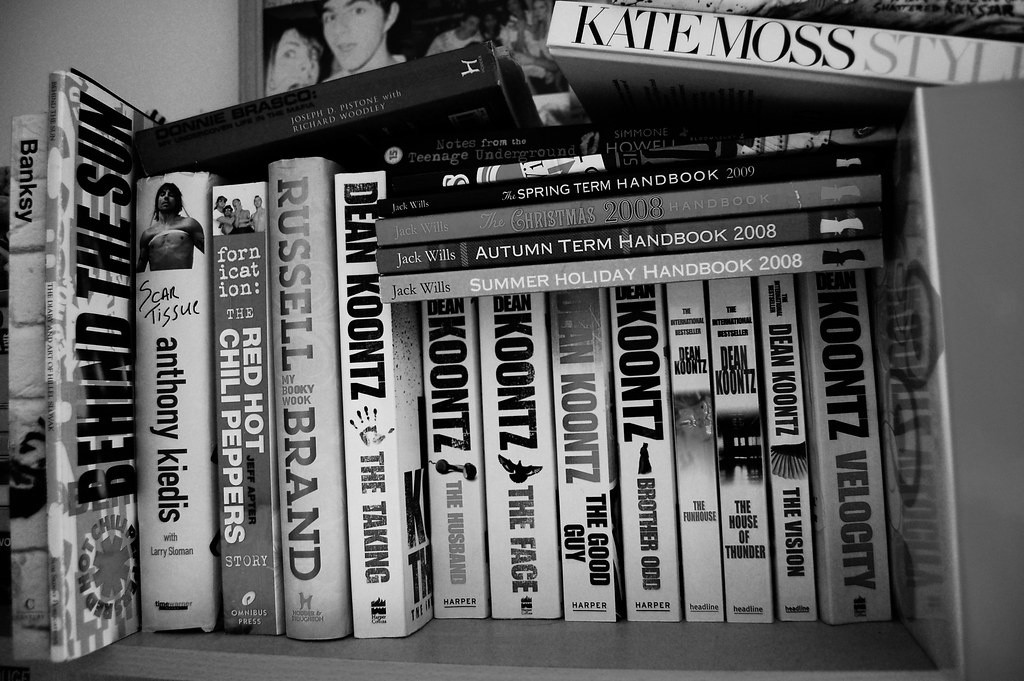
[137,183,205,273]
[319,0,406,82]
[213,196,265,235]
[501,0,560,81]
[427,13,484,56]
[263,16,329,99]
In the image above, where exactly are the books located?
[335,170,434,642]
[376,119,959,626]
[132,167,223,633]
[45,70,156,666]
[7,112,48,669]
[207,181,282,640]
[545,2,1024,91]
[127,53,551,177]
[269,155,351,642]
